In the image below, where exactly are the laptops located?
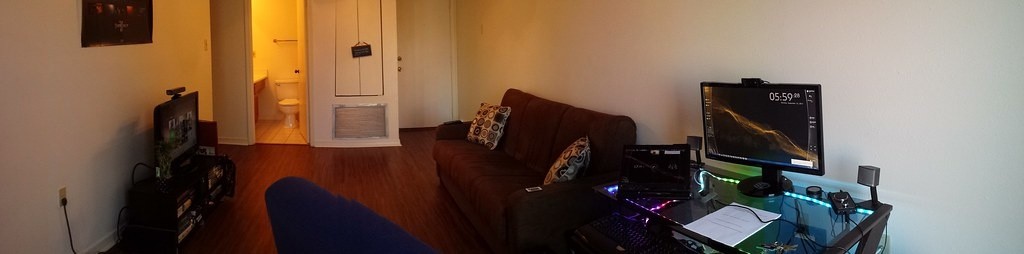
[616,144,694,200]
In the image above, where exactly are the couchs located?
[265,176,439,254]
[432,89,636,254]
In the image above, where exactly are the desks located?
[570,160,892,254]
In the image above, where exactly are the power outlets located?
[59,187,66,208]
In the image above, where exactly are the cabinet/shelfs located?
[126,154,228,254]
[254,78,268,123]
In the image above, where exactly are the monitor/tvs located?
[700,81,825,197]
[154,91,199,174]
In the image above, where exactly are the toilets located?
[274,79,299,128]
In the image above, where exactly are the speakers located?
[686,136,705,167]
[855,166,883,211]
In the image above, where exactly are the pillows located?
[544,137,591,185]
[466,102,512,151]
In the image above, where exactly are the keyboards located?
[575,211,699,254]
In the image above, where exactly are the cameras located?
[828,189,857,215]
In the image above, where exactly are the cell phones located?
[525,186,542,192]
